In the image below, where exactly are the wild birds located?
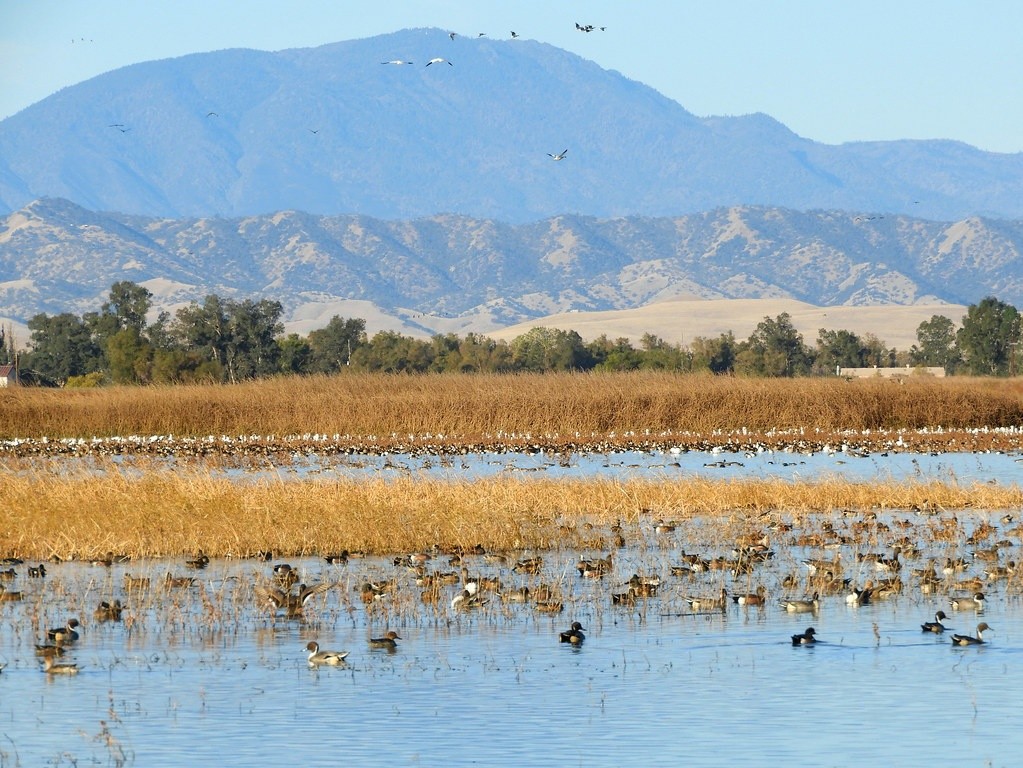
[478,32,488,37]
[380,60,413,64]
[108,124,131,133]
[509,31,519,39]
[0,424,1023,474]
[426,58,454,67]
[206,113,219,118]
[546,148,569,160]
[306,128,319,135]
[575,21,606,33]
[448,32,458,40]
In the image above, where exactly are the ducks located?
[0,497,1022,676]
